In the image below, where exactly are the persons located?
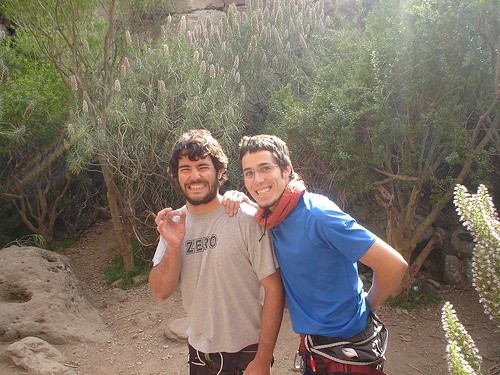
[149,129,285,375]
[222,134,408,375]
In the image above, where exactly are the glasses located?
[240,163,279,178]
